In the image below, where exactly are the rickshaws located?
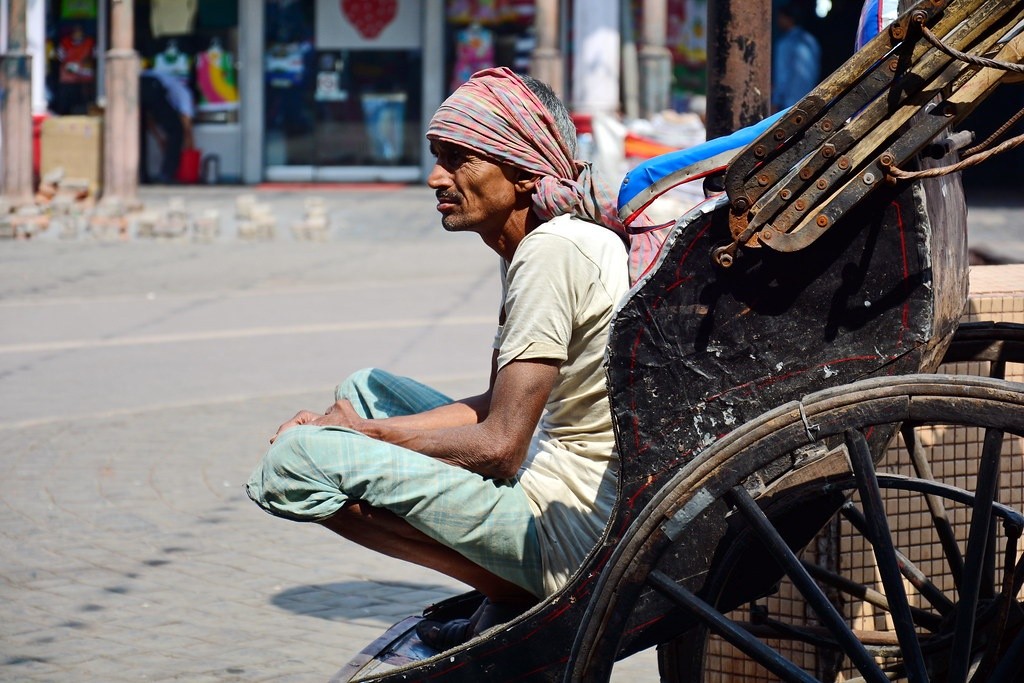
[332,1,1024,683]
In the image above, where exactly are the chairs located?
[334,124,969,683]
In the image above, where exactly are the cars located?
[141,102,285,182]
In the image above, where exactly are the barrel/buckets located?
[178,149,200,183]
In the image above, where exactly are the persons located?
[247,66,634,654]
[771,3,822,115]
[140,74,193,182]
[55,18,237,115]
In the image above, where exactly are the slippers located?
[415,618,474,651]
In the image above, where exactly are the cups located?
[204,155,217,183]
